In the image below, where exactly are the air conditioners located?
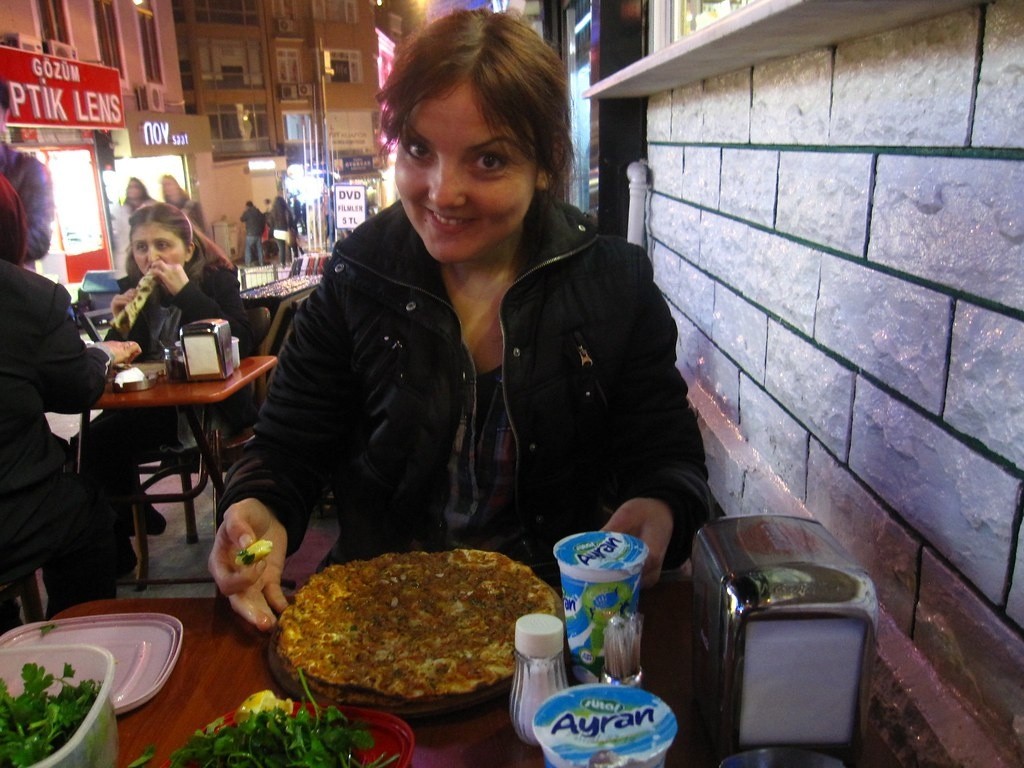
[135,82,166,112]
[7,32,44,54]
[45,38,79,60]
[276,19,293,33]
[280,85,298,100]
[298,84,313,96]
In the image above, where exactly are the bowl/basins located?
[0,644,119,768]
[159,703,414,768]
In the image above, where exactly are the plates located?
[267,578,569,719]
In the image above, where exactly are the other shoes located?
[116,506,167,537]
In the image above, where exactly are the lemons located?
[233,689,282,724]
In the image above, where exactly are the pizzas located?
[273,546,557,702]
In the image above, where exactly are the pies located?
[108,267,161,336]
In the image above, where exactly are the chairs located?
[0,270,316,636]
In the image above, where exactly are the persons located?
[80,202,259,580]
[240,201,266,267]
[118,177,154,277]
[266,194,306,269]
[209,8,726,634]
[0,174,141,639]
[161,174,205,232]
[0,80,51,270]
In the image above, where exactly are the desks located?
[89,356,279,594]
[43,575,908,768]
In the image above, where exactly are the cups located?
[232,337,240,369]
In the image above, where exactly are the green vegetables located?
[0,621,117,768]
[118,664,403,768]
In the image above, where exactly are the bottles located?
[164,347,182,381]
[509,614,569,746]
